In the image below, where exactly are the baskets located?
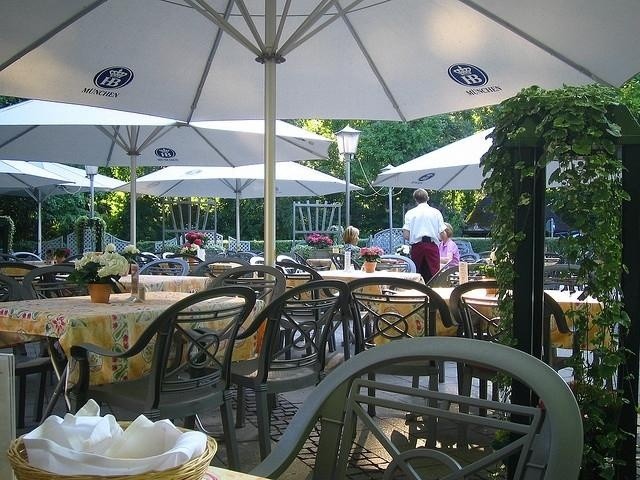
[6,420,217,480]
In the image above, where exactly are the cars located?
[462,226,492,236]
[548,227,581,237]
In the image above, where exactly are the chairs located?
[0,232,618,480]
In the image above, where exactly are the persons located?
[439,223,460,268]
[402,188,447,284]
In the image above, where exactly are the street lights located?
[84,164,100,250]
[380,162,396,253]
[335,123,363,230]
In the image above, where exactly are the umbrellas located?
[374,129,622,190]
[0,99,336,250]
[1,0,640,298]
